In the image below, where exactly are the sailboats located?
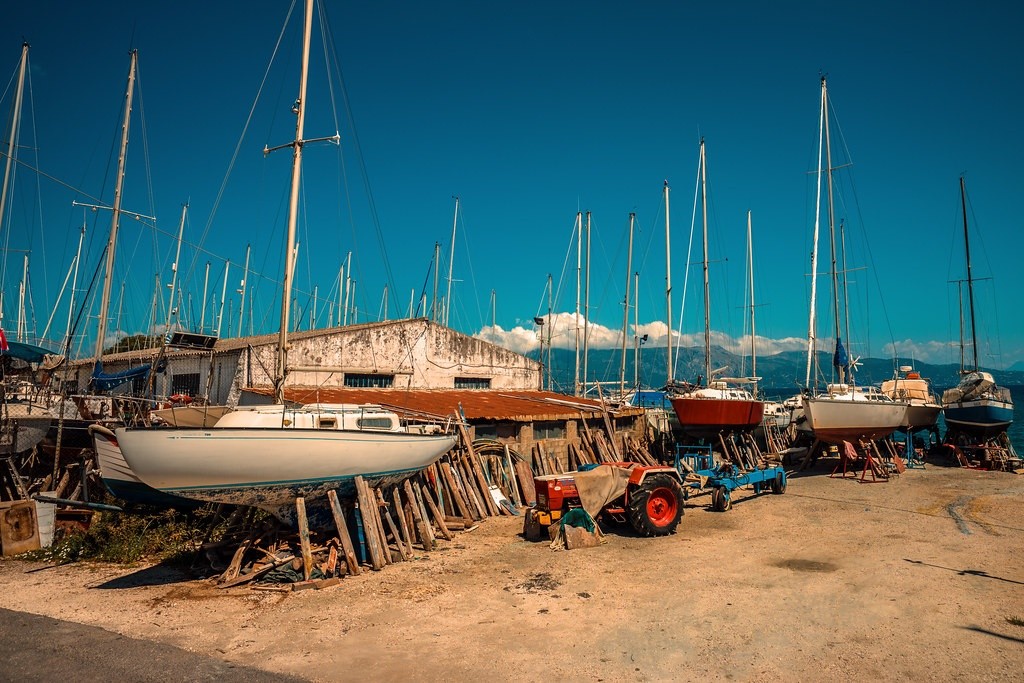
[801,76,912,442]
[114,0,460,516]
[4,0,785,492]
[668,132,765,429]
[941,176,1015,439]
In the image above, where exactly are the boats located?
[880,365,940,434]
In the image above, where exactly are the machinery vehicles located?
[524,443,788,541]
